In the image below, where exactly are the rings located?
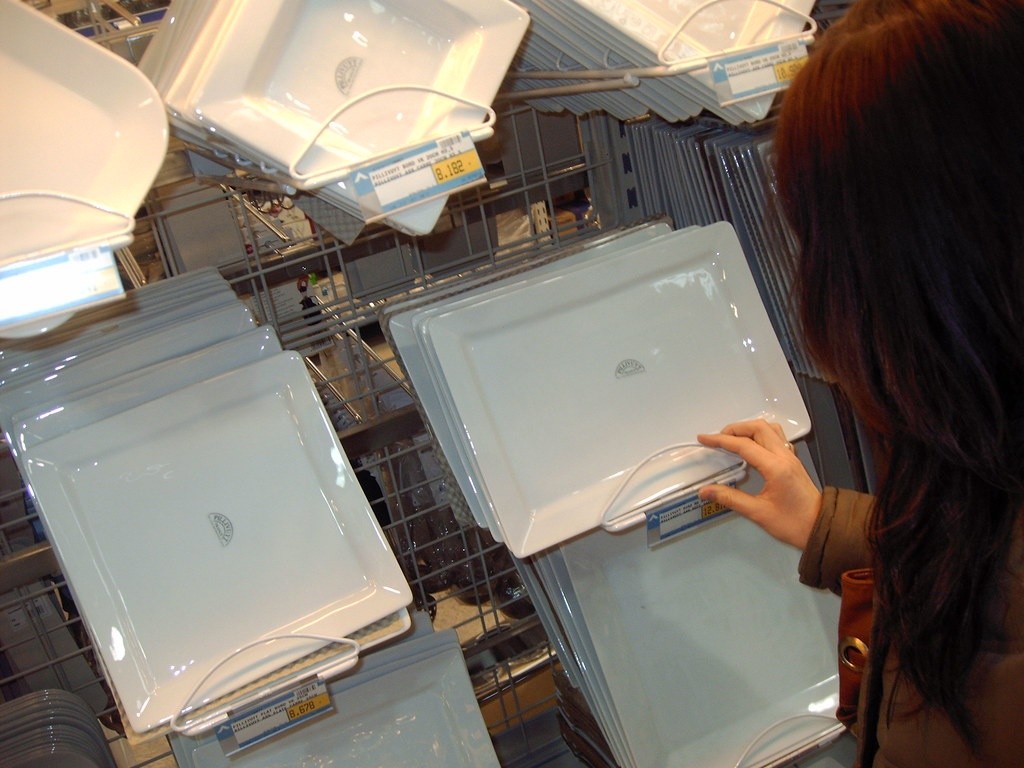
[784,444,791,449]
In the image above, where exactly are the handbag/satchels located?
[835,569,879,724]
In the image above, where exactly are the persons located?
[697,0,1024,768]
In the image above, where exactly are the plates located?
[1,0,844,768]
